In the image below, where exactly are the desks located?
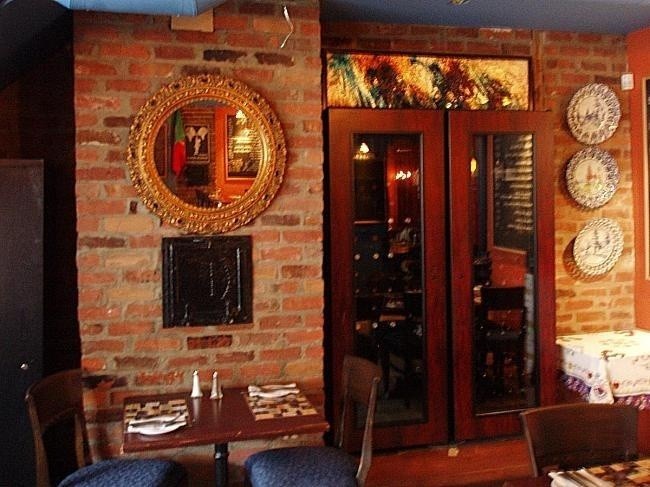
[557,328,650,409]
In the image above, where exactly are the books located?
[546,459,650,487]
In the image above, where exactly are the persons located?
[184,126,201,156]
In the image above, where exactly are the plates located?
[574,218,625,276]
[138,428,177,436]
[261,394,290,400]
[565,82,621,210]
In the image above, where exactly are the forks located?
[130,415,188,427]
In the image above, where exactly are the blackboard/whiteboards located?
[487,130,535,254]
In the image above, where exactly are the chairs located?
[474,286,531,403]
[25,368,187,487]
[519,402,639,477]
[356,288,425,395]
[244,356,384,486]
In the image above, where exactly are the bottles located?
[189,370,223,401]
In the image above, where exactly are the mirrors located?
[125,74,289,236]
